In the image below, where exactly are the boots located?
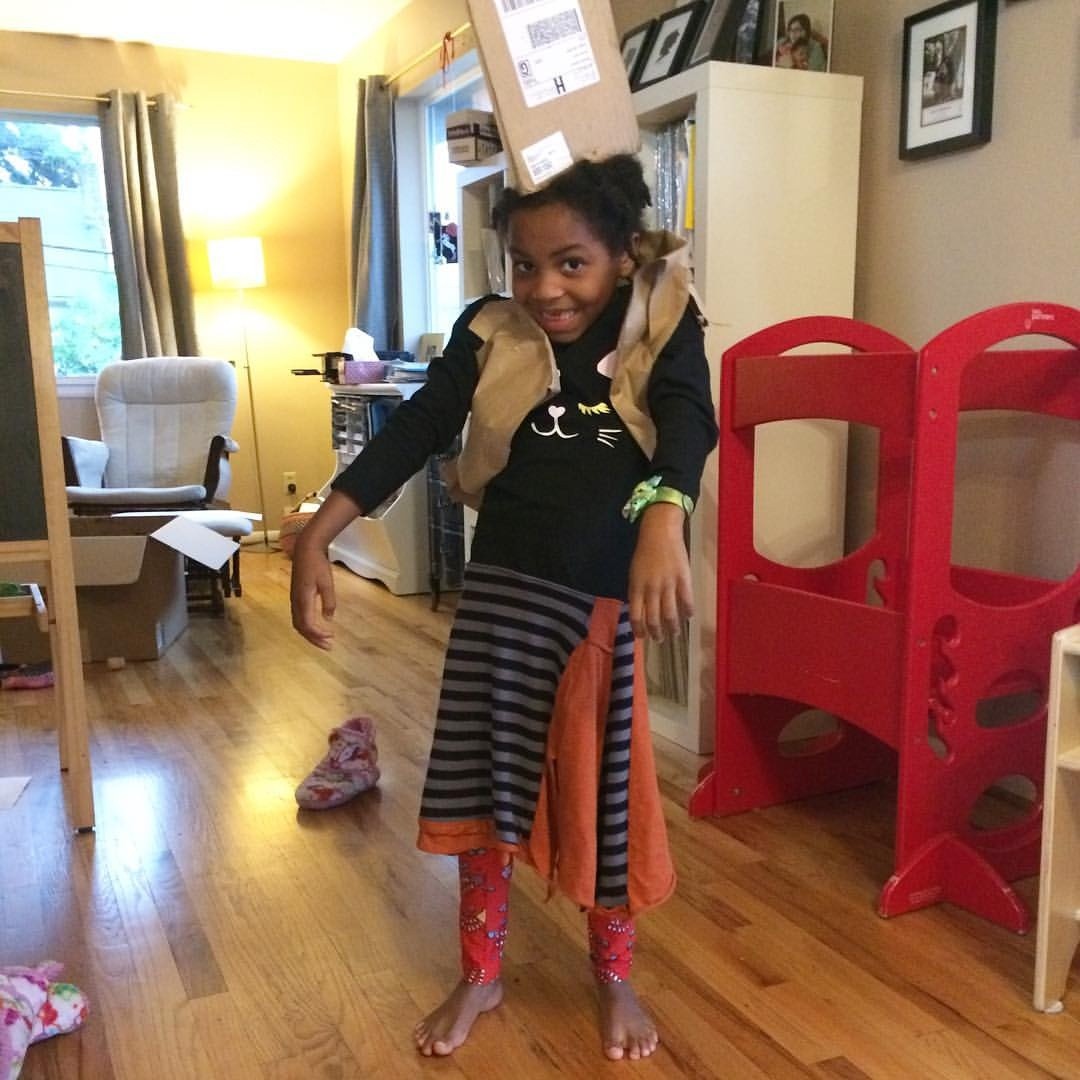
[295,717,381,809]
[0,960,89,1080]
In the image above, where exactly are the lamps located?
[207,236,287,553]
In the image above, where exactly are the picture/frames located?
[772,1,834,71]
[898,0,999,160]
[685,0,740,69]
[617,19,655,94]
[635,1,705,85]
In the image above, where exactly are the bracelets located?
[621,475,697,525]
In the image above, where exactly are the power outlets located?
[284,473,297,495]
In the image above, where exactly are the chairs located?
[62,353,247,620]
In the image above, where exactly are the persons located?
[286,153,722,1064]
[775,13,829,74]
[923,33,966,107]
[650,29,681,71]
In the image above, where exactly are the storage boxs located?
[444,108,499,165]
[65,513,238,661]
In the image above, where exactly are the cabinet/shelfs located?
[292,371,466,597]
[462,55,870,751]
[1030,626,1080,1012]
